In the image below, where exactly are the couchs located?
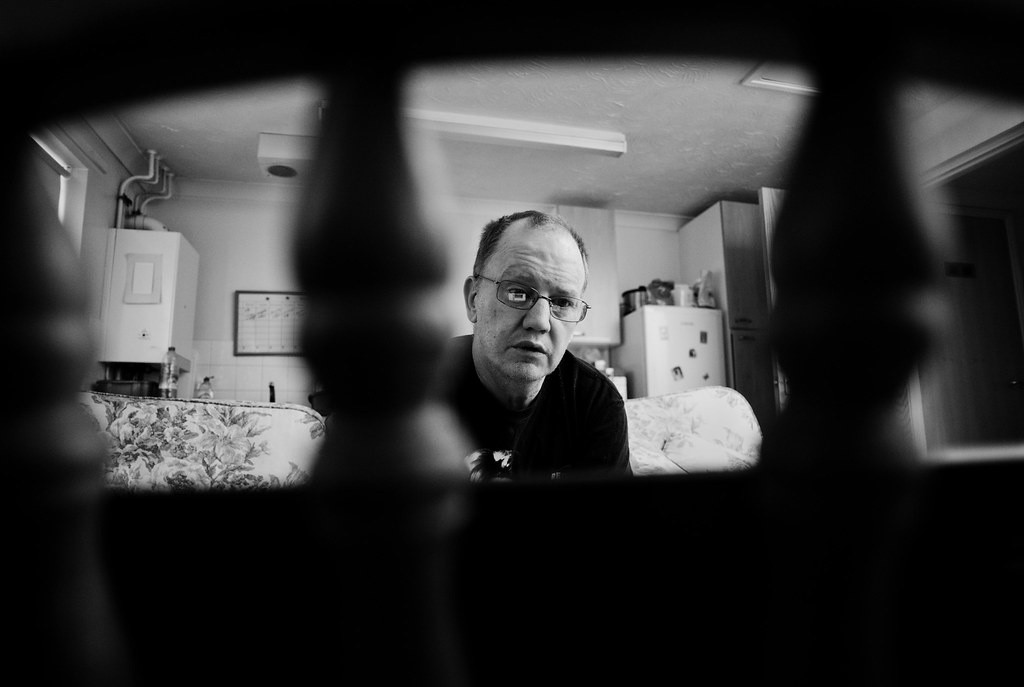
[78,385,765,493]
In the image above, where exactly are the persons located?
[309,211,633,484]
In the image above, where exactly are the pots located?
[621,285,649,314]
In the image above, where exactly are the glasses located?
[474,274,592,322]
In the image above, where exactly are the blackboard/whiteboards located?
[232,291,310,356]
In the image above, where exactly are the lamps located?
[403,108,628,159]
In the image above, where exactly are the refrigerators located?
[611,304,727,397]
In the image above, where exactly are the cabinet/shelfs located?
[676,200,775,419]
[549,205,621,346]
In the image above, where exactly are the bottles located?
[161,346,178,398]
[196,377,215,400]
